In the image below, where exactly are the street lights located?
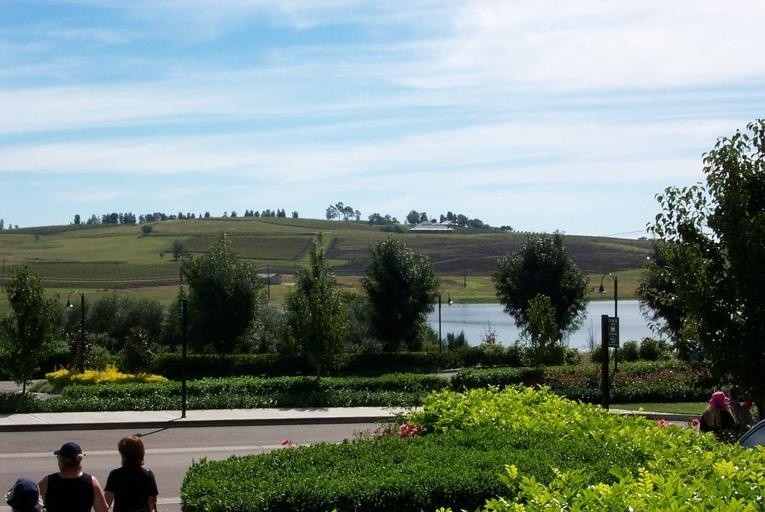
[66,290,85,372]
[438,289,453,353]
[598,272,620,373]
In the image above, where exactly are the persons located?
[36,441,111,511]
[102,435,159,511]
[699,390,737,444]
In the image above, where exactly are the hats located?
[54,442,81,456]
[709,391,731,406]
